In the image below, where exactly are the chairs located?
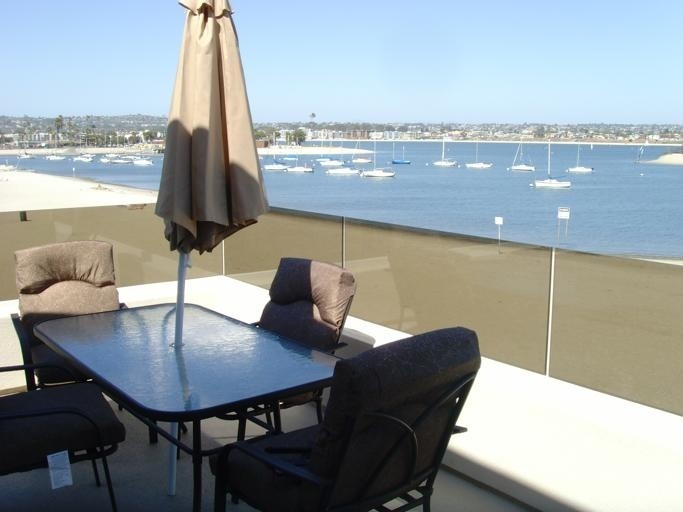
[213,256,356,443]
[7,240,158,443]
[1,382,125,510]
[210,326,478,512]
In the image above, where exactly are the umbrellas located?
[154,0,268,497]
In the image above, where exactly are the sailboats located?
[533,138,572,189]
[464,138,493,169]
[563,146,594,174]
[432,142,457,167]
[264,136,411,178]
[16,134,155,168]
[510,137,536,171]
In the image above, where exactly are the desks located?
[34,302,336,508]
